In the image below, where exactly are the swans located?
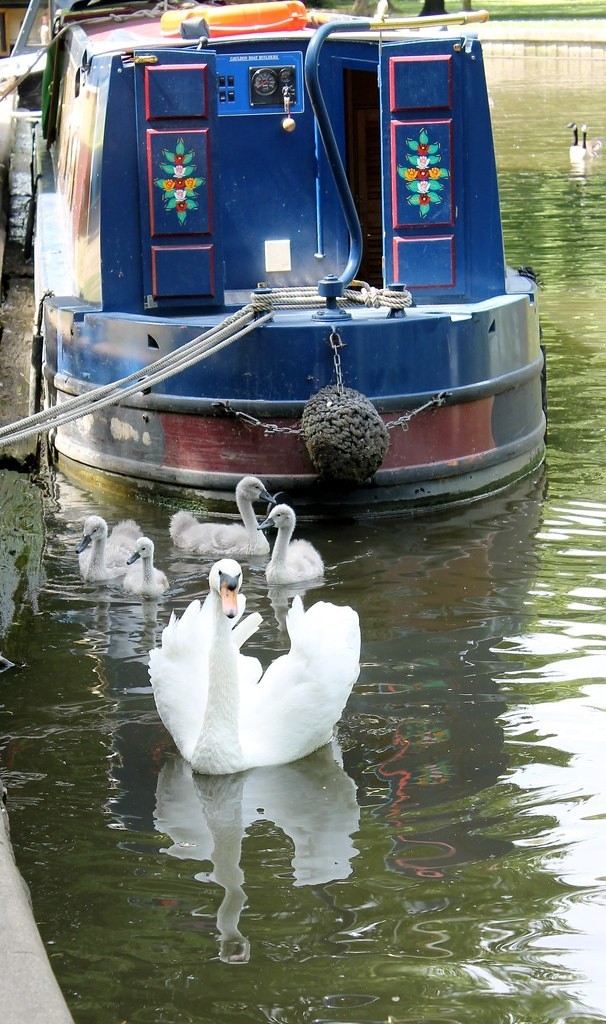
[565,122,604,165]
[76,474,366,779]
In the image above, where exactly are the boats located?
[28,10,549,535]
